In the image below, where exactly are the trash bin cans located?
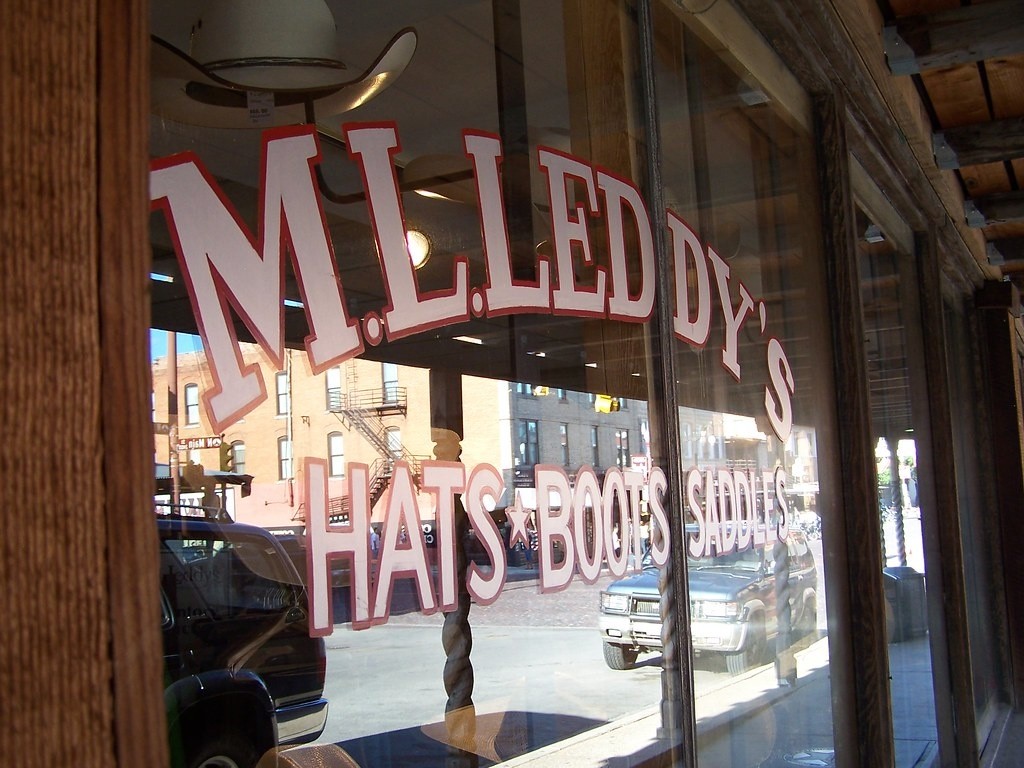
[883,566,929,640]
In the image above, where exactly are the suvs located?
[157,499,329,747]
[598,519,819,676]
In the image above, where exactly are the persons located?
[522,530,534,570]
[774,506,783,525]
[611,520,649,557]
[791,506,801,527]
[371,528,379,559]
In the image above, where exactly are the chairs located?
[735,548,762,571]
